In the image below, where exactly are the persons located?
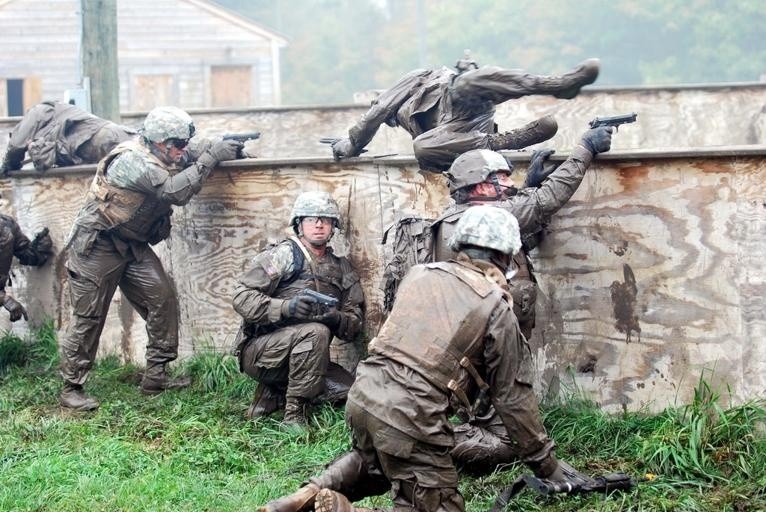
[0,211,51,325]
[330,58,598,173]
[0,100,260,179]
[231,191,366,427]
[260,204,571,512]
[57,106,247,410]
[429,127,619,466]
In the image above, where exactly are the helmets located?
[448,204,523,257]
[142,105,196,147]
[446,147,512,194]
[287,190,345,230]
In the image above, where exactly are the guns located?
[319,139,347,161]
[29,228,51,267]
[304,288,339,316]
[521,460,633,502]
[223,132,260,145]
[588,112,637,133]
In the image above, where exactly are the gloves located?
[2,295,29,323]
[524,146,557,188]
[313,303,341,330]
[579,125,614,154]
[30,234,53,254]
[198,138,244,169]
[281,293,316,321]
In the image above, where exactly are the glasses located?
[169,137,192,150]
[297,216,335,226]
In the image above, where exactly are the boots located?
[532,58,600,102]
[487,114,557,152]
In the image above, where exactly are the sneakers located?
[141,366,191,393]
[59,380,102,413]
[255,476,383,511]
[247,381,315,446]
